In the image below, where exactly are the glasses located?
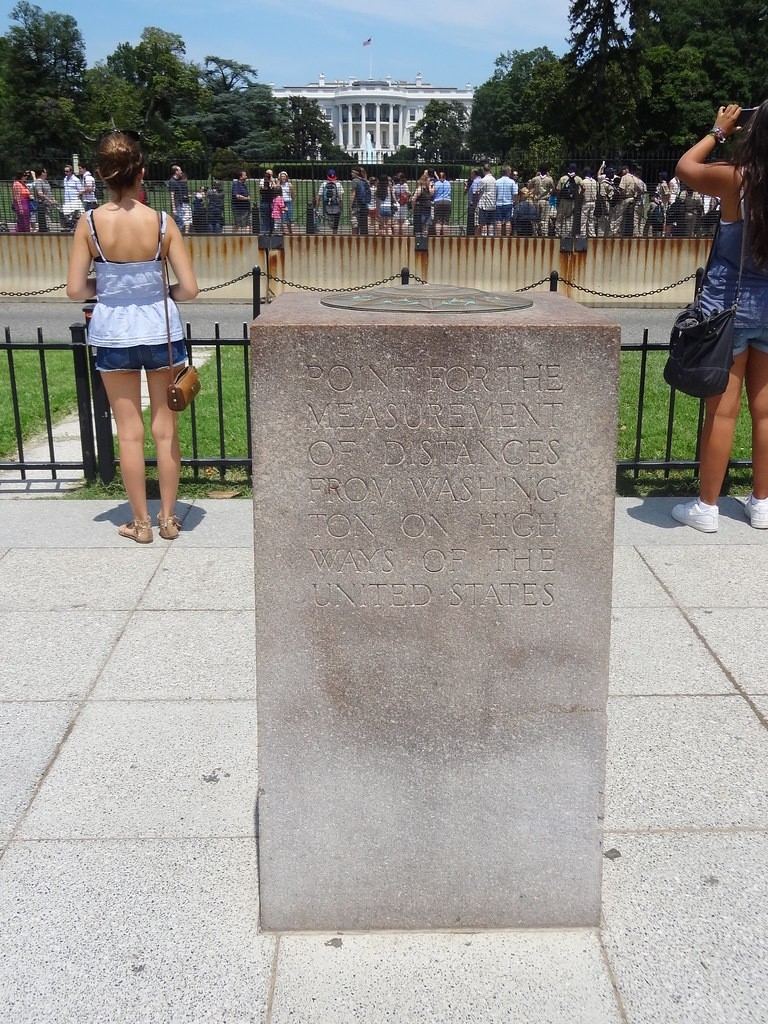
[22,175,26,176]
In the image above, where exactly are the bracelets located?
[710,126,726,143]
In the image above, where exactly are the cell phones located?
[735,108,757,127]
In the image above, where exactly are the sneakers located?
[671,496,719,534]
[744,492,768,530]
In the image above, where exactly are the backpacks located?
[322,182,340,205]
[607,189,620,207]
[651,204,664,230]
[562,174,577,200]
[84,175,104,200]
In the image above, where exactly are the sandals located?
[118,517,153,544]
[158,510,181,540]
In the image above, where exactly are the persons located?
[12,160,720,240]
[663,101,768,533]
[67,126,202,544]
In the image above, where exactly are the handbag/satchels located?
[172,214,185,233]
[167,364,201,412]
[663,297,739,398]
[399,192,407,205]
[594,193,603,217]
[391,197,398,213]
[29,199,37,211]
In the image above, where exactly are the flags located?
[363,37,371,47]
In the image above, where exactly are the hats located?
[569,163,576,170]
[327,169,336,178]
[658,171,668,179]
[605,167,617,175]
